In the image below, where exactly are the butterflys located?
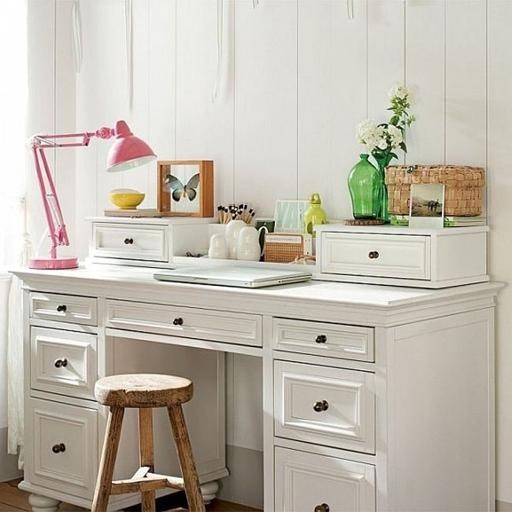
[164,172,201,204]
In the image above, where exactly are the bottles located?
[347,154,382,220]
[303,193,327,238]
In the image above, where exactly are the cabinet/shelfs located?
[305,223,494,289]
[82,211,224,266]
[8,260,504,512]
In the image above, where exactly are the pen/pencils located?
[217,203,256,224]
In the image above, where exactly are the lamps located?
[28,118,158,269]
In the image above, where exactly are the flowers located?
[357,82,416,156]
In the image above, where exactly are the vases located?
[350,153,401,221]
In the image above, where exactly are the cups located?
[207,220,269,262]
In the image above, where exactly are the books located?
[389,214,484,222]
[391,220,485,227]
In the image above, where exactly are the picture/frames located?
[158,158,215,216]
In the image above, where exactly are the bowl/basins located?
[109,193,146,210]
